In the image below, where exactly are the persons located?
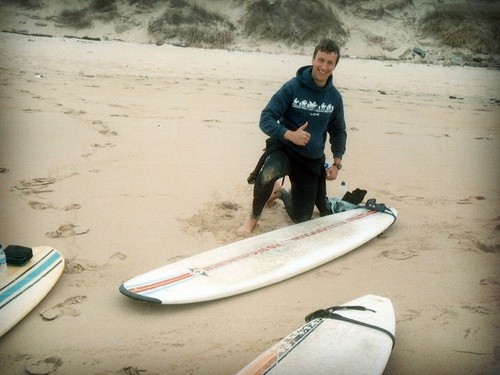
[236,38,347,237]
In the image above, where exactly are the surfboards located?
[236,288,396,375]
[119,198,398,307]
[0,241,67,337]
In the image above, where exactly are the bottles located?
[324,162,332,169]
[0,244,8,277]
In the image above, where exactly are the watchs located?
[333,163,342,170]
